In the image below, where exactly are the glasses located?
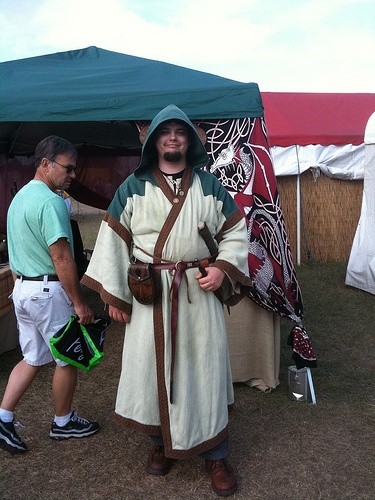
[50,159,80,174]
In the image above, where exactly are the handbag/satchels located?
[128,263,156,305]
[49,313,112,371]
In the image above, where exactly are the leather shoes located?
[146,442,173,476]
[205,457,238,495]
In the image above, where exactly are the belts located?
[16,274,60,281]
[131,252,220,404]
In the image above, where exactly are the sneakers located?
[0,417,29,455]
[50,409,100,441]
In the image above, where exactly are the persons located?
[0,135,99,453]
[80,103,247,496]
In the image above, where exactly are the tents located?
[258,92,374,266]
[0,46,318,403]
